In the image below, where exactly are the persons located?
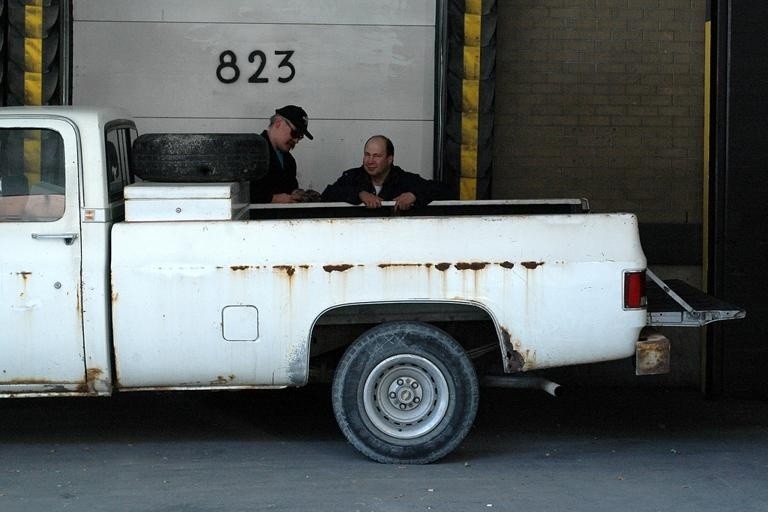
[249,104,320,204]
[321,133,438,214]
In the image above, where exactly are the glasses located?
[284,120,305,139]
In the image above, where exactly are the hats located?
[275,105,313,140]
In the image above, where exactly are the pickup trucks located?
[0,104,748,471]
